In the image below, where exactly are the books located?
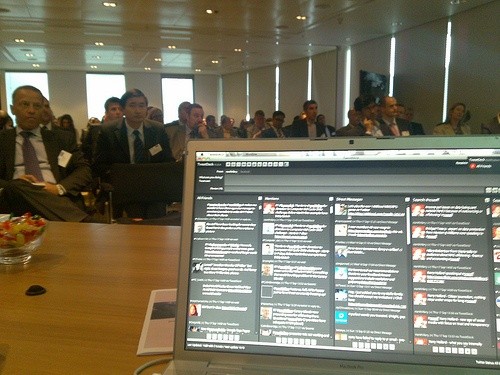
[136,287,178,356]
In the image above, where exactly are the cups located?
[0,214,11,222]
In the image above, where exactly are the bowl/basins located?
[0,217,48,264]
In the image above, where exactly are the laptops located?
[163,135,499,375]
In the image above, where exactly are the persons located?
[163,102,191,127]
[317,114,335,138]
[189,304,199,317]
[0,86,107,223]
[92,88,172,220]
[238,117,274,139]
[53,114,81,147]
[145,106,163,122]
[432,103,472,135]
[283,116,301,137]
[290,100,325,140]
[0,111,13,133]
[335,94,425,136]
[104,97,123,120]
[39,97,71,131]
[87,113,106,125]
[262,111,294,138]
[204,115,218,131]
[165,103,213,166]
[212,115,239,138]
[247,110,270,138]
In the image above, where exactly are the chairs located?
[109,160,184,225]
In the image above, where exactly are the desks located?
[0,219,182,375]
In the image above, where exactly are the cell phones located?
[32,182,46,188]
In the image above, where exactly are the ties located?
[278,129,282,138]
[389,123,399,137]
[19,131,44,183]
[133,130,147,168]
[43,126,48,129]
[323,126,328,138]
[310,122,315,137]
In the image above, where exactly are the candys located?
[0,212,48,251]
